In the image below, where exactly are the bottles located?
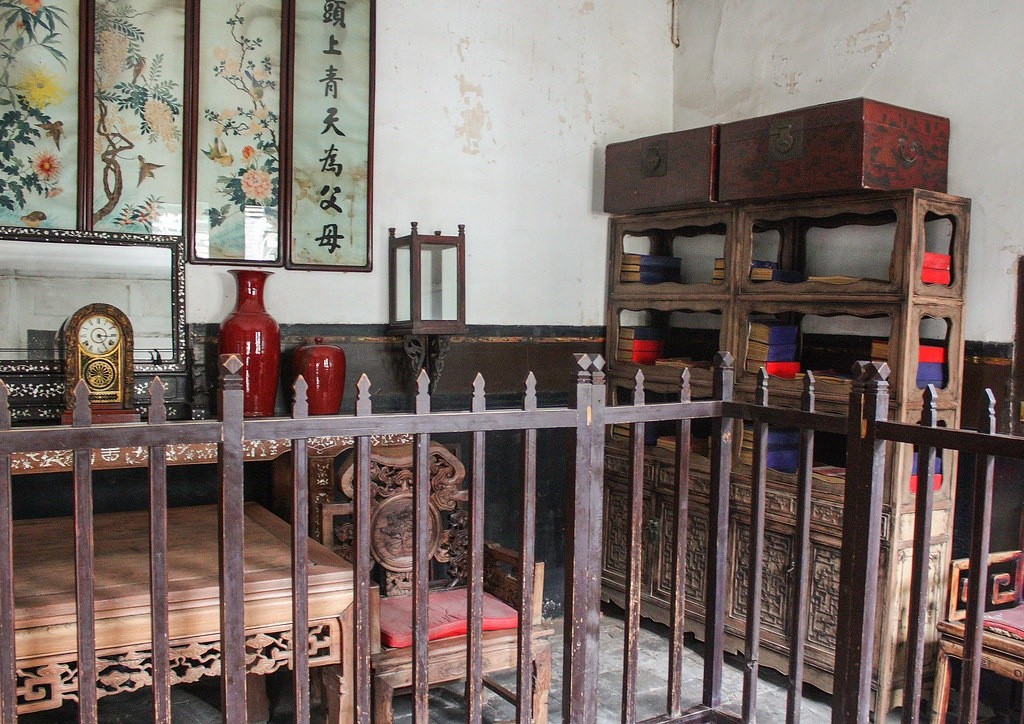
[219,269,282,418]
[292,337,347,416]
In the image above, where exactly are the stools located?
[369,539,555,724]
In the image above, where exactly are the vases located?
[292,337,346,414]
[218,269,281,418]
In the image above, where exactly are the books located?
[811,465,846,483]
[708,424,800,474]
[794,369,855,385]
[656,357,715,370]
[621,254,681,283]
[712,258,777,285]
[909,452,944,492]
[889,250,951,285]
[656,435,709,455]
[746,319,800,378]
[613,421,668,446]
[808,276,891,285]
[617,327,673,365]
[871,341,949,389]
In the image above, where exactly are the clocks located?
[62,303,142,424]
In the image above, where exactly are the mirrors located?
[0,226,190,422]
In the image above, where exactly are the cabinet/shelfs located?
[597,186,973,714]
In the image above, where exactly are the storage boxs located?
[717,96,952,203]
[387,221,466,329]
[602,124,720,215]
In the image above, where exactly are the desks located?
[12,501,354,724]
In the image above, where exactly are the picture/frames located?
[0,0,376,274]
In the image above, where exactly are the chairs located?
[921,510,1024,724]
